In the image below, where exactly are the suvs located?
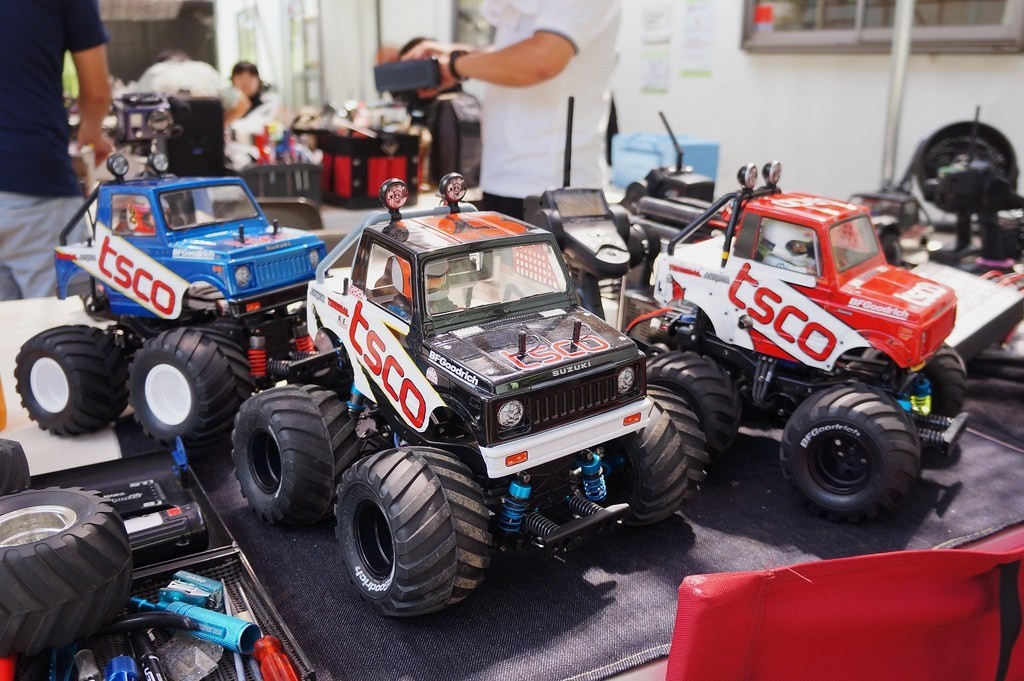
[838,181,934,269]
[618,159,972,527]
[230,173,713,623]
[13,149,349,465]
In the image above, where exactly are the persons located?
[136,49,223,177]
[230,61,282,123]
[0,0,114,300]
[400,1,621,221]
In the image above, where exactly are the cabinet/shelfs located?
[741,0,1024,55]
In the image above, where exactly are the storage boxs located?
[611,131,719,186]
[311,126,420,210]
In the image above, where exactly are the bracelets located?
[449,50,469,81]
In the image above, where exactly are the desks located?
[0,243,1024,681]
